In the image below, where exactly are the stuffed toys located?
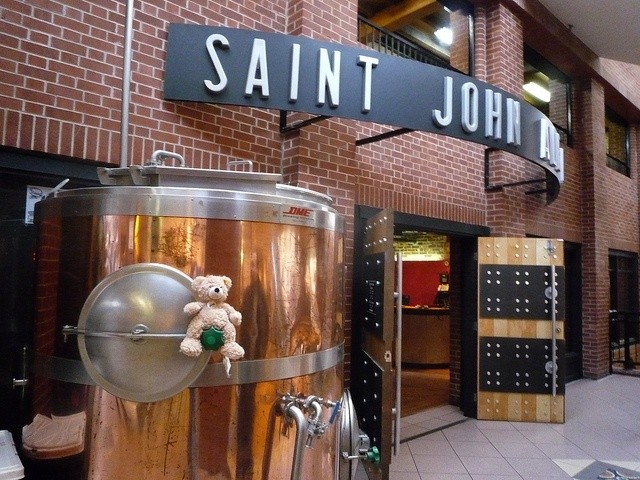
[179,274,246,361]
